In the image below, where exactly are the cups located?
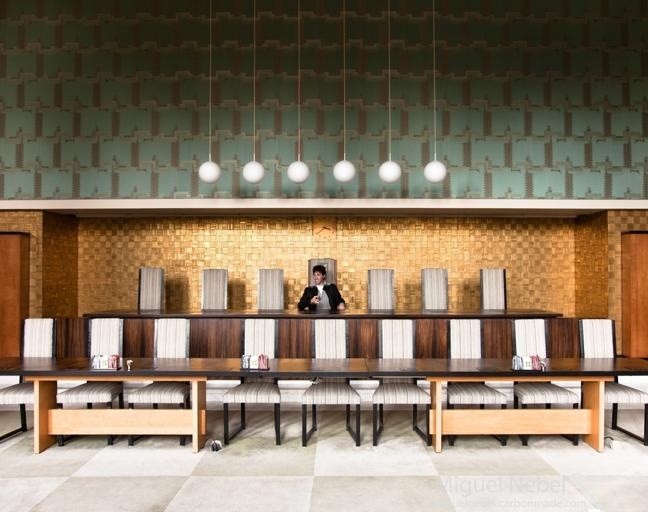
[90,356,120,368]
[512,353,548,371]
[127,361,137,372]
[241,355,269,368]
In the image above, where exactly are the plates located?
[239,368,270,372]
[510,367,542,372]
[88,368,122,373]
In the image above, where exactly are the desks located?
[81,309,563,359]
[0,358,647,454]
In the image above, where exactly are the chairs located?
[420,268,447,309]
[301,319,360,447]
[137,267,163,309]
[368,269,395,309]
[578,318,647,446]
[512,319,578,446]
[0,318,55,442]
[56,318,124,447]
[201,269,227,310]
[308,259,337,286]
[480,269,506,309]
[223,319,281,445]
[128,318,189,446]
[256,268,283,310]
[372,319,431,447]
[446,319,506,446]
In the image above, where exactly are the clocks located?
[311,215,337,242]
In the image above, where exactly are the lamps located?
[332,0,355,182]
[286,0,309,183]
[198,0,220,183]
[423,0,445,182]
[377,0,401,183]
[242,0,264,183]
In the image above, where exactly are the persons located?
[297,265,345,311]
